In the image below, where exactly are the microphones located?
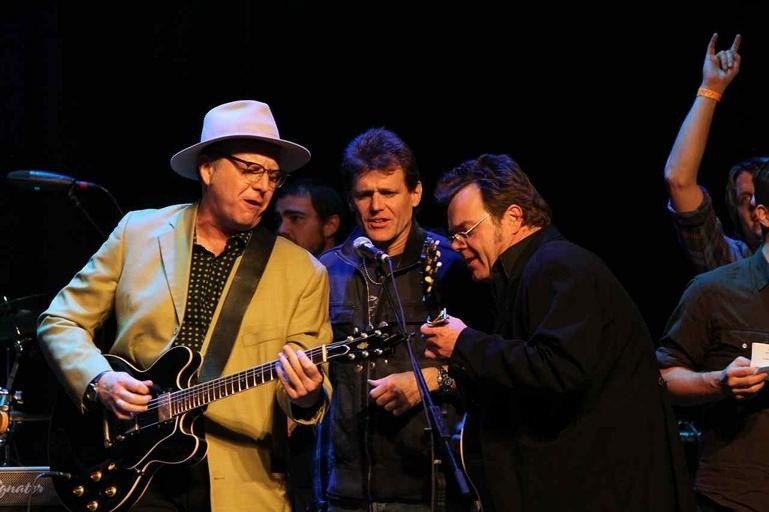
[8,168,78,186]
[351,237,392,265]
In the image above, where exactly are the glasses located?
[448,212,491,242]
[225,154,286,187]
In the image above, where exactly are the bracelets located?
[697,86,723,104]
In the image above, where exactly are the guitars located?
[50,321,406,509]
[421,238,524,511]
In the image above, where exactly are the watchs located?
[84,369,112,404]
[433,363,456,395]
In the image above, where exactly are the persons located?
[276,178,344,257]
[45,96,332,511]
[316,128,474,511]
[663,30,769,276]
[651,159,769,511]
[418,157,696,511]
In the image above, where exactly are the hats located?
[171,100,311,180]
